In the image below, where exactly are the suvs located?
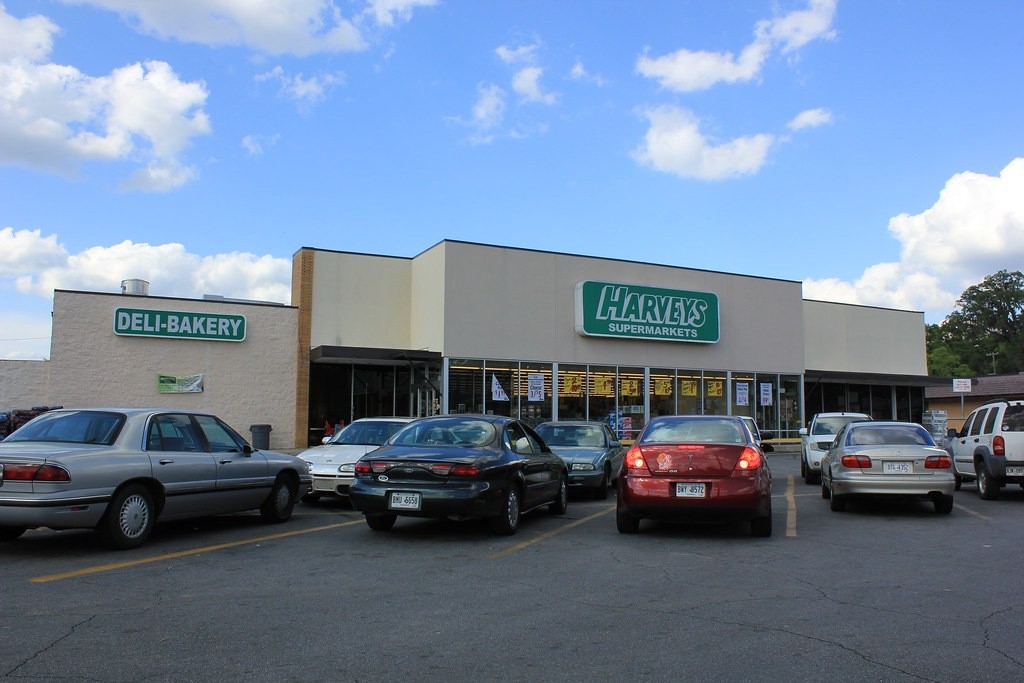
[943,398,1024,501]
[800,412,875,487]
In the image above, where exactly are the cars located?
[291,418,419,506]
[740,416,775,449]
[821,420,956,518]
[1,407,312,554]
[519,421,625,503]
[617,416,775,538]
[348,413,570,540]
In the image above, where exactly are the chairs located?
[161,436,186,450]
[429,425,453,445]
